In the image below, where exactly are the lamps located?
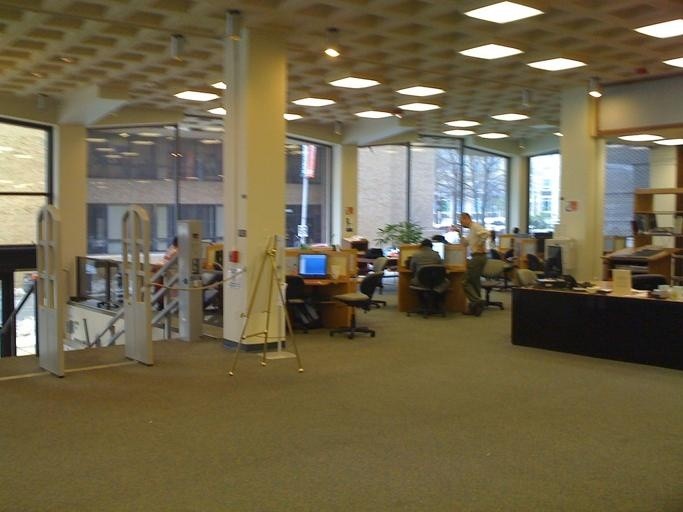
[397,102,440,111]
[395,86,447,97]
[457,44,525,60]
[588,77,602,98]
[442,130,476,136]
[444,120,481,127]
[618,134,663,142]
[477,132,510,139]
[653,139,683,145]
[224,10,239,39]
[328,76,381,89]
[324,27,340,57]
[283,114,304,120]
[174,91,221,102]
[491,114,530,121]
[354,110,393,119]
[662,58,683,68]
[291,97,336,106]
[169,35,185,61]
[463,1,545,24]
[632,18,683,39]
[526,57,587,71]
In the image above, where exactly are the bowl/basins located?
[593,286,602,292]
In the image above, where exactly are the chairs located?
[517,269,539,286]
[633,275,665,290]
[490,248,520,291]
[480,259,504,311]
[369,249,383,259]
[527,254,543,270]
[407,265,449,319]
[357,257,386,311]
[330,272,384,339]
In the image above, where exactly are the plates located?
[573,288,587,292]
[598,289,614,293]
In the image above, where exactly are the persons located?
[485,229,515,263]
[407,239,442,286]
[163,235,177,264]
[451,212,491,317]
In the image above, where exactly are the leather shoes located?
[475,299,485,316]
[463,309,475,315]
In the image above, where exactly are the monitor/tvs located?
[433,242,446,261]
[299,254,328,277]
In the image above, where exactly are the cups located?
[659,286,669,299]
[332,266,340,280]
[670,287,683,302]
[586,288,598,294]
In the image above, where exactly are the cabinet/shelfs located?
[544,239,575,270]
[633,189,683,286]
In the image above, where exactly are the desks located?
[511,288,683,370]
[600,246,682,285]
